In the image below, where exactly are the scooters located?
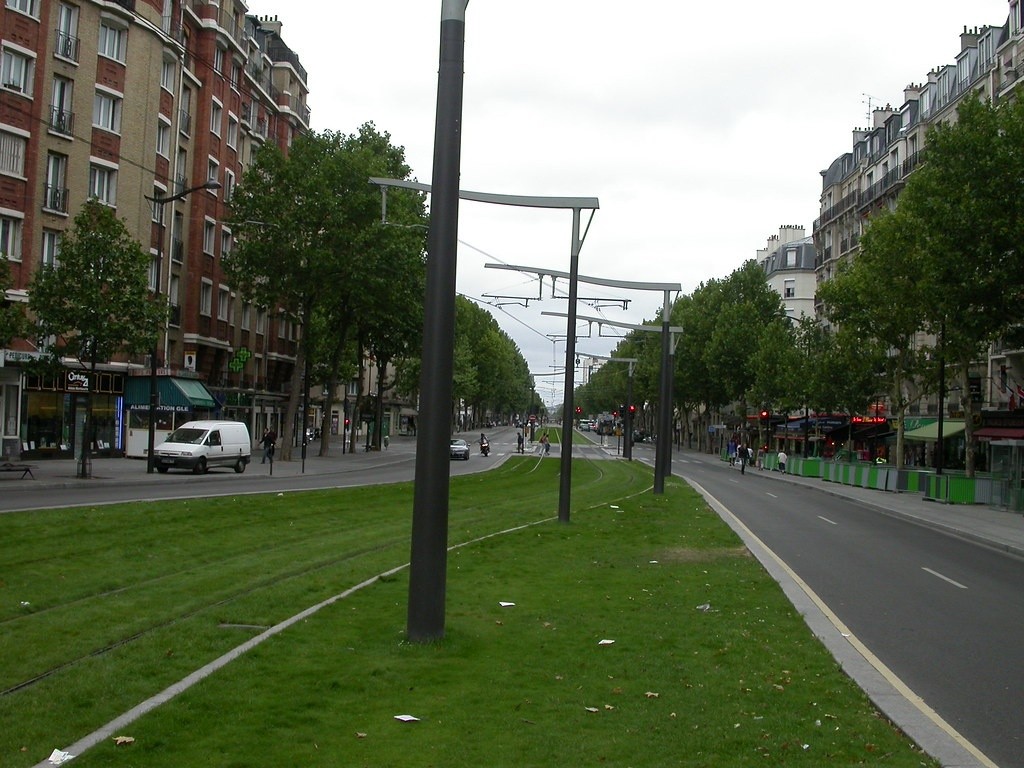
[478,439,491,457]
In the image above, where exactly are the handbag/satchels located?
[539,436,544,443]
[267,445,275,458]
[735,457,742,466]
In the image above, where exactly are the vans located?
[153,419,251,475]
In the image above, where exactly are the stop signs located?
[529,415,536,422]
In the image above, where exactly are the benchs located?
[0,464,39,480]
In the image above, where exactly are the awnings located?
[904,421,966,442]
[971,427,1024,439]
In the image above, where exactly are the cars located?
[306,426,322,445]
[449,438,471,460]
[579,415,653,443]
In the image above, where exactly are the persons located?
[257,426,278,464]
[728,439,788,474]
[479,433,551,456]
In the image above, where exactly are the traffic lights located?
[630,404,636,411]
[576,406,582,414]
[760,410,769,418]
[345,418,350,428]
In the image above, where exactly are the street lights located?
[145,180,222,474]
